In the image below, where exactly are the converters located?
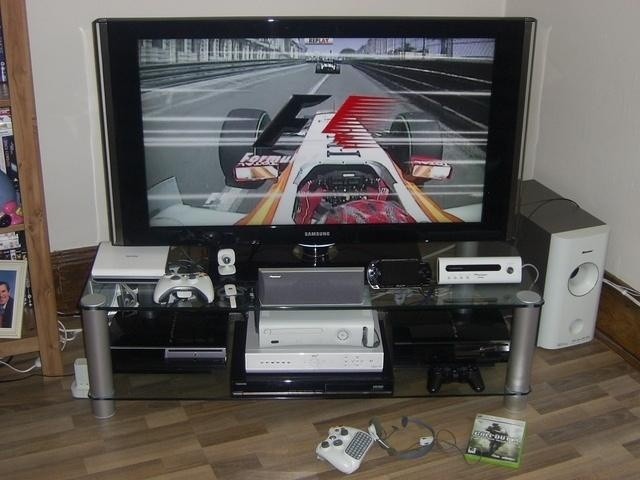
[74,358,89,387]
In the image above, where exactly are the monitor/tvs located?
[91,15,539,270]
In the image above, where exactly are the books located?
[463,413,531,469]
[0,108,33,308]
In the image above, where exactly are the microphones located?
[367,430,397,458]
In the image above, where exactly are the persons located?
[486,423,508,456]
[0,282,13,328]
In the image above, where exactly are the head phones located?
[367,413,436,461]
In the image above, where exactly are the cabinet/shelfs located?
[0,1,66,393]
[75,239,541,420]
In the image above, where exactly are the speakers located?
[511,179,611,350]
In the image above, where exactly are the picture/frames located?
[1,258,29,341]
